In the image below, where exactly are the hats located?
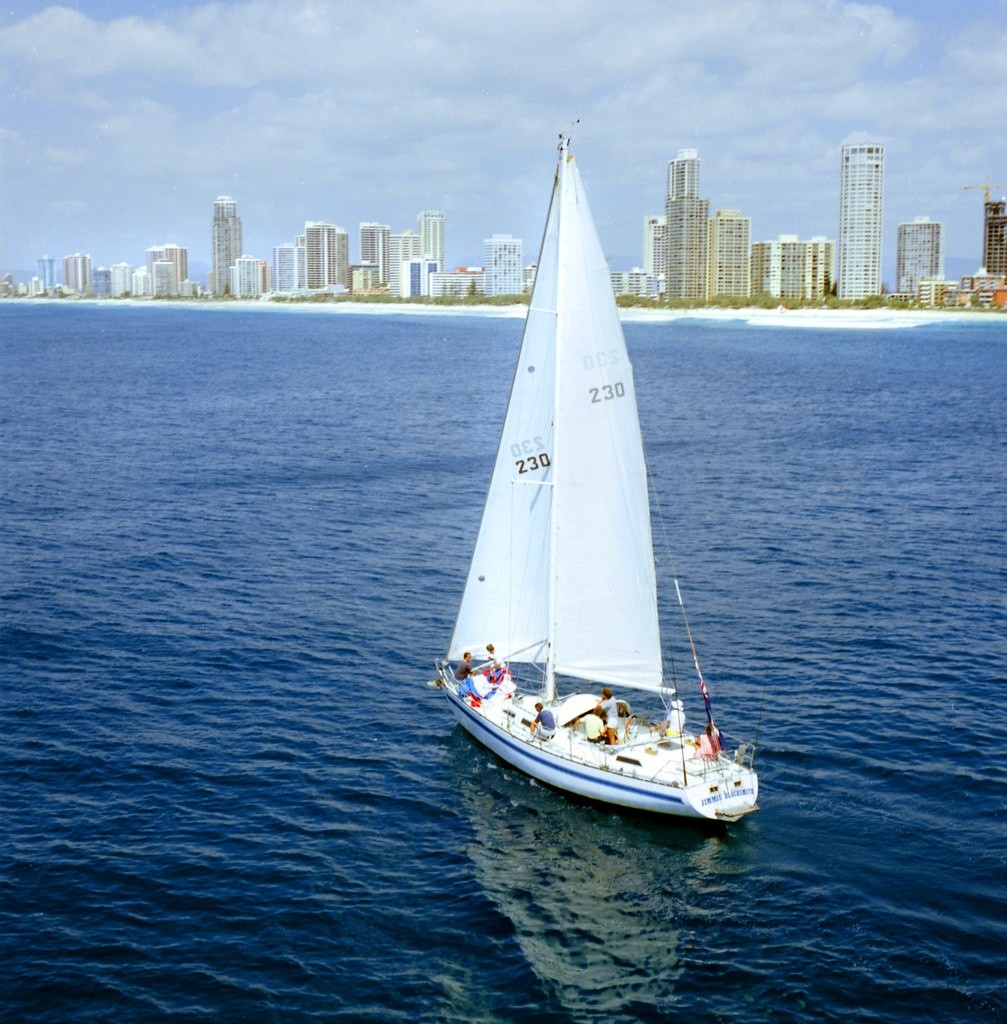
[671,700,684,712]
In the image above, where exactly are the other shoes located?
[527,738,534,742]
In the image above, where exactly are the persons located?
[486,644,505,669]
[573,688,619,745]
[527,703,555,742]
[694,725,718,761]
[454,652,476,681]
[626,715,637,743]
[649,700,685,736]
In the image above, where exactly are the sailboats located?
[432,130,761,831]
